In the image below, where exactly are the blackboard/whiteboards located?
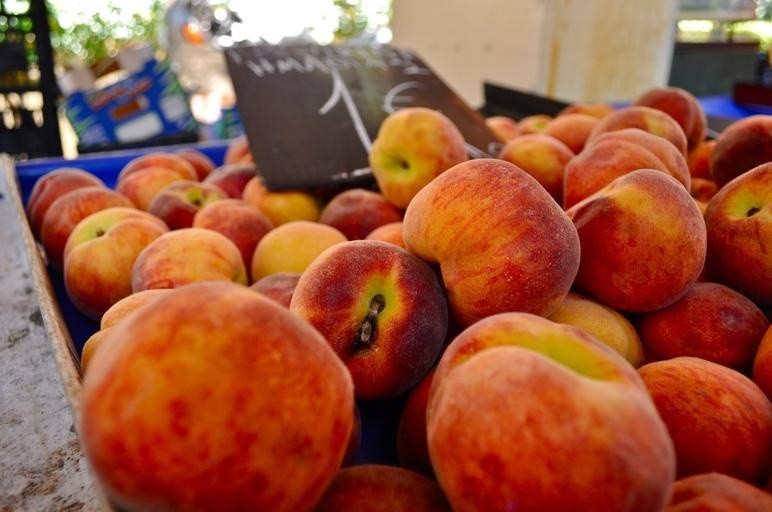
[222,46,508,192]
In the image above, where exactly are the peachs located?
[31,84,772,512]
